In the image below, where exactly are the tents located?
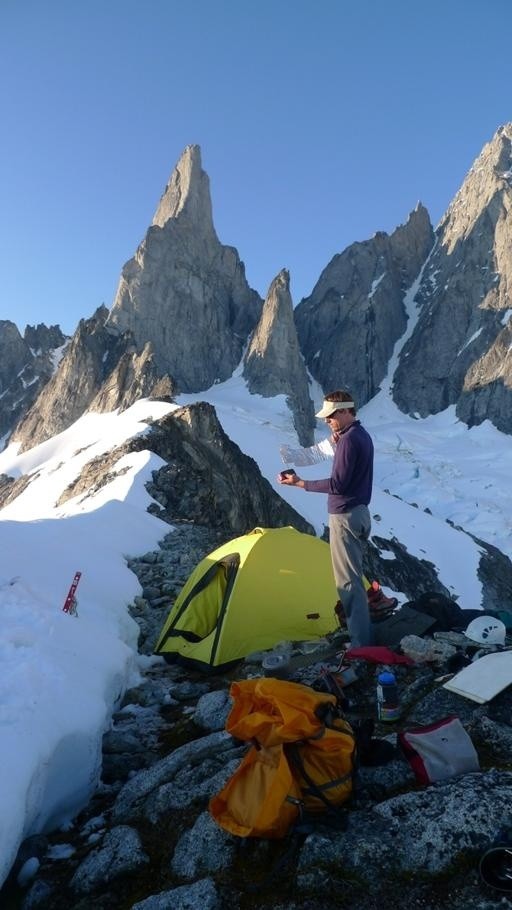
[154,525,371,668]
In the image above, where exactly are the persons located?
[278,390,375,652]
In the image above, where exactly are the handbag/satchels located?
[209,677,357,841]
[398,715,481,785]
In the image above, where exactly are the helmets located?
[464,615,506,647]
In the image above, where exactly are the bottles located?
[261,653,291,681]
[376,671,403,724]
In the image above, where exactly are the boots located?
[367,587,398,615]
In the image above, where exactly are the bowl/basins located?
[478,847,512,893]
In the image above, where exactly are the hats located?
[314,400,355,418]
